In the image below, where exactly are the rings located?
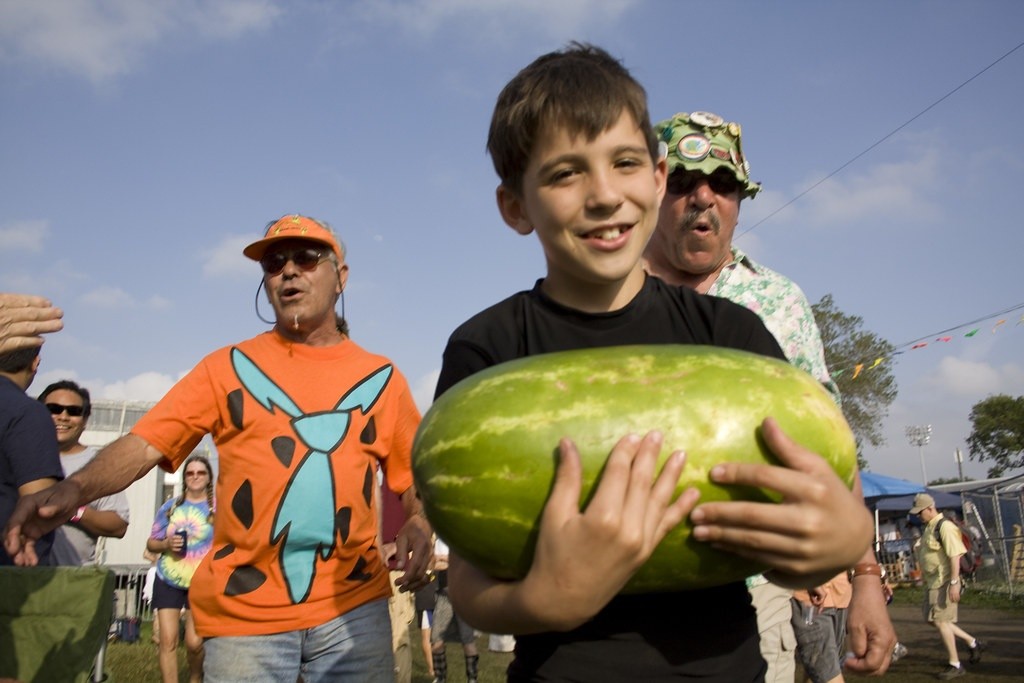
[427,570,437,580]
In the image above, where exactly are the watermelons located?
[409,342,859,594]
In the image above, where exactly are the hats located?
[910,494,934,514]
[651,111,761,201]
[243,216,342,264]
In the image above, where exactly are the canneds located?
[174,527,188,557]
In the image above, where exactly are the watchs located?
[950,576,961,585]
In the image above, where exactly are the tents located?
[861,472,1024,560]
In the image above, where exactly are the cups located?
[175,529,186,555]
[801,599,819,625]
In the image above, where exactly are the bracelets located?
[70,503,86,525]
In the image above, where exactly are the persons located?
[1,214,437,683]
[0,278,991,683]
[431,38,898,683]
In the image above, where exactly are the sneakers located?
[891,645,906,663]
[938,662,965,681]
[968,638,988,664]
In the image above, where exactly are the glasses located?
[261,248,338,277]
[46,402,84,417]
[184,469,208,476]
[665,171,738,195]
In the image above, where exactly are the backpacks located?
[934,519,983,576]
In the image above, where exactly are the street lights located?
[904,423,935,486]
